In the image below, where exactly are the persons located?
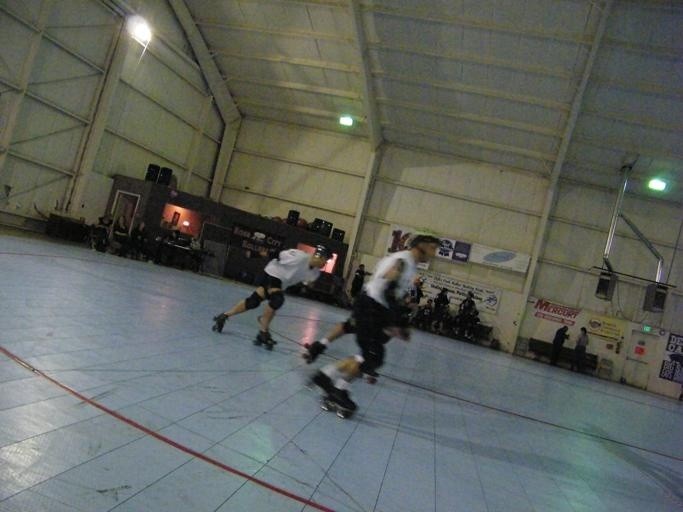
[215,243,336,348]
[550,326,570,366]
[130,220,153,262]
[311,234,443,412]
[350,262,372,303]
[570,326,589,373]
[411,287,481,342]
[300,278,428,378]
[91,213,114,251]
[114,215,130,257]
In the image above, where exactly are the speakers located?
[145,163,173,187]
[311,217,345,242]
[595,271,617,302]
[286,210,300,226]
[643,283,669,313]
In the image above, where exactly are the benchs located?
[528,337,597,370]
[478,324,492,340]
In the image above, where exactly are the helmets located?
[410,234,441,249]
[314,244,333,259]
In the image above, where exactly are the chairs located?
[597,358,614,381]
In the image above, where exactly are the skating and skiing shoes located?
[356,359,380,384]
[252,329,277,351]
[212,312,228,333]
[302,369,357,419]
[302,341,327,364]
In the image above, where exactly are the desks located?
[47,213,91,245]
[172,244,193,271]
